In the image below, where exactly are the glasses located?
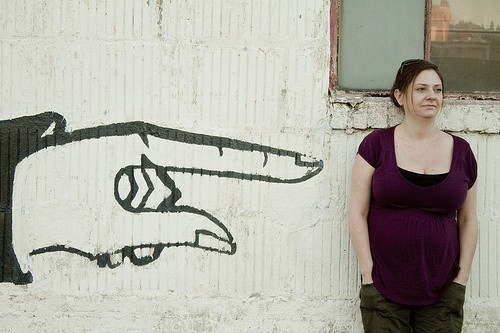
[401,59,421,74]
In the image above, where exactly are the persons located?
[348,59,479,333]
